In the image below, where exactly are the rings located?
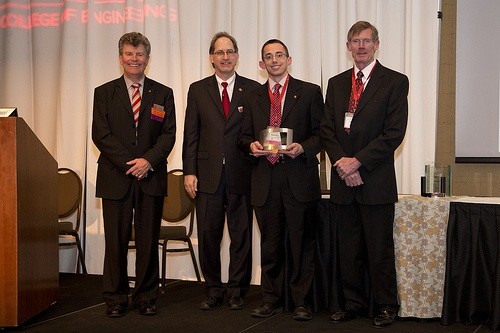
[137,172,140,175]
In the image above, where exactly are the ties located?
[221,81,231,121]
[265,84,282,165]
[345,71,364,135]
[130,83,142,145]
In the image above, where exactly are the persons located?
[182,32,261,310]
[92,32,177,318]
[238,39,324,319]
[320,21,409,328]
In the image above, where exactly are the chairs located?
[54,168,89,277]
[159,169,201,288]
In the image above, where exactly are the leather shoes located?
[106,302,128,316]
[250,302,283,317]
[139,300,159,314]
[292,305,313,321]
[228,294,261,312]
[199,294,224,310]
[372,312,397,328]
[328,308,357,323]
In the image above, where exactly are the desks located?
[278,194,500,324]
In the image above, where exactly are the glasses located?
[264,52,289,61]
[350,37,377,46]
[213,49,237,57]
